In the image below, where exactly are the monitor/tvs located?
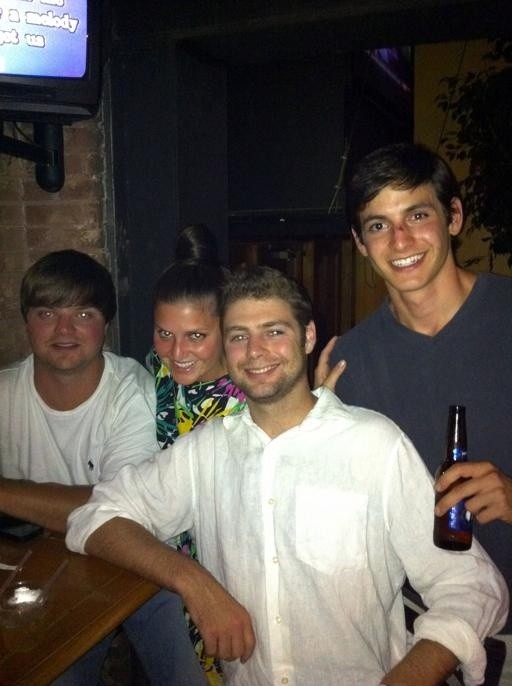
[0,1,105,112]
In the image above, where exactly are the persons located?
[0,247,162,533]
[64,265,509,686]
[326,141,511,686]
[134,260,341,562]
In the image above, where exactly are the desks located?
[1,512,168,686]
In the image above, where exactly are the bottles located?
[431,404,478,553]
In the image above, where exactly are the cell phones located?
[1,510,44,543]
